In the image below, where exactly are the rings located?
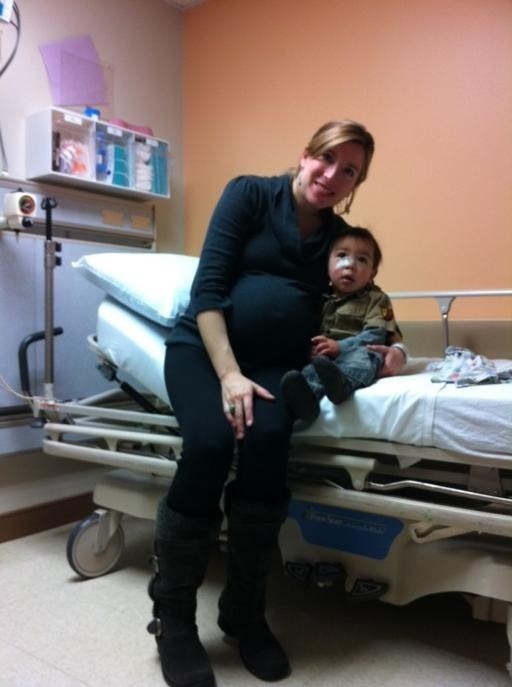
[230,405,235,415]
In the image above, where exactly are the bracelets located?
[390,342,411,364]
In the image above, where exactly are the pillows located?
[70,251,201,328]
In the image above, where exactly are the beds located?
[37,289,512,679]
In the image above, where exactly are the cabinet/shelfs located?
[24,105,172,199]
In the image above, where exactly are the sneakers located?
[310,353,351,405]
[281,370,319,422]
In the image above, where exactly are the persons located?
[148,119,409,687]
[279,228,397,422]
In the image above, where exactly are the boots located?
[218,480,291,680]
[148,495,223,687]
